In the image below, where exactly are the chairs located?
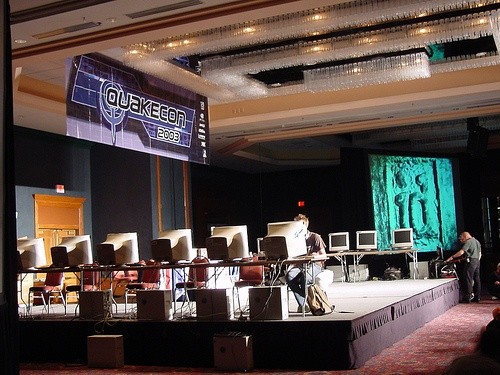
[65,264,101,316]
[174,256,210,315]
[125,259,159,314]
[29,264,65,315]
[233,257,266,316]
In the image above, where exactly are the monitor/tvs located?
[356,230,378,251]
[262,220,307,260]
[51,234,93,269]
[151,229,192,265]
[16,237,47,270]
[392,228,413,250]
[206,223,248,262]
[329,232,349,252]
[96,231,139,267]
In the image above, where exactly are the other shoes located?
[313,307,325,316]
[470,297,480,302]
[459,299,469,304]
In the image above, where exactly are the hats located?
[458,233,463,239]
[493,307,500,317]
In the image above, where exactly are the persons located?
[5,245,19,310]
[430,248,443,268]
[286,214,327,314]
[444,232,482,302]
[490,262,500,300]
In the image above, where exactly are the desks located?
[17,258,326,319]
[327,252,346,283]
[345,249,419,283]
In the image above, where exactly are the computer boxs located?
[87,334,124,368]
[249,286,288,320]
[349,264,368,282]
[326,265,345,282]
[213,335,253,370]
[79,290,111,319]
[195,288,230,321]
[136,290,171,320]
[409,261,428,279]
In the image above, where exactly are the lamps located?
[124,0,500,103]
